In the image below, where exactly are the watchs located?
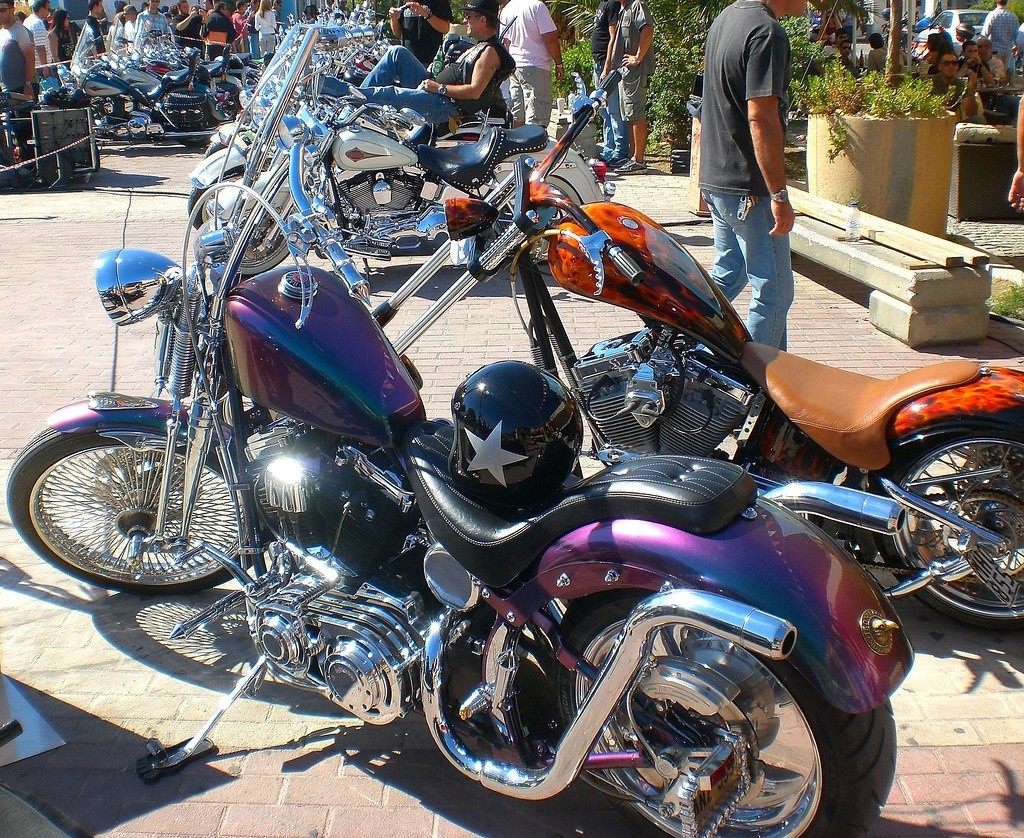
[768,190,789,203]
[438,84,447,95]
[423,12,433,20]
[977,63,984,68]
[24,80,33,86]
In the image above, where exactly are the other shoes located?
[606,158,622,167]
[596,157,607,162]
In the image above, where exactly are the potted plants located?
[789,50,970,241]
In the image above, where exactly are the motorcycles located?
[369,68,1024,632]
[5,27,915,838]
[36,0,630,279]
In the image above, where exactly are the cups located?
[557,98,564,113]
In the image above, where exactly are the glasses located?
[0,6,13,11]
[463,12,479,20]
[150,0,158,2]
[940,60,957,66]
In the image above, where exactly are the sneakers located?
[609,158,630,170]
[614,156,647,175]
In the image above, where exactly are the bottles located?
[845,202,861,241]
[432,45,444,78]
[568,90,576,114]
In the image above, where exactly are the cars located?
[914,9,1018,64]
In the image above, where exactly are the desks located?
[978,86,1022,111]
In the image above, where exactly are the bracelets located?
[555,63,563,68]
[634,55,640,65]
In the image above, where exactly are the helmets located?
[447,359,582,513]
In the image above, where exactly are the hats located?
[458,0,506,27]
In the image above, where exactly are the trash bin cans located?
[689,73,711,216]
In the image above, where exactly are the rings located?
[415,9,418,12]
[1017,193,1022,198]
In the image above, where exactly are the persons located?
[292,0,516,125]
[388,0,453,71]
[698,0,809,352]
[0,0,37,177]
[499,0,564,129]
[303,0,334,22]
[923,32,1007,124]
[818,10,840,51]
[979,0,1020,89]
[589,0,655,176]
[822,28,908,77]
[1007,94,1024,214]
[15,0,285,87]
[1016,20,1024,70]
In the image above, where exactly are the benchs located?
[947,141,1024,224]
[546,109,597,159]
[785,184,992,349]
